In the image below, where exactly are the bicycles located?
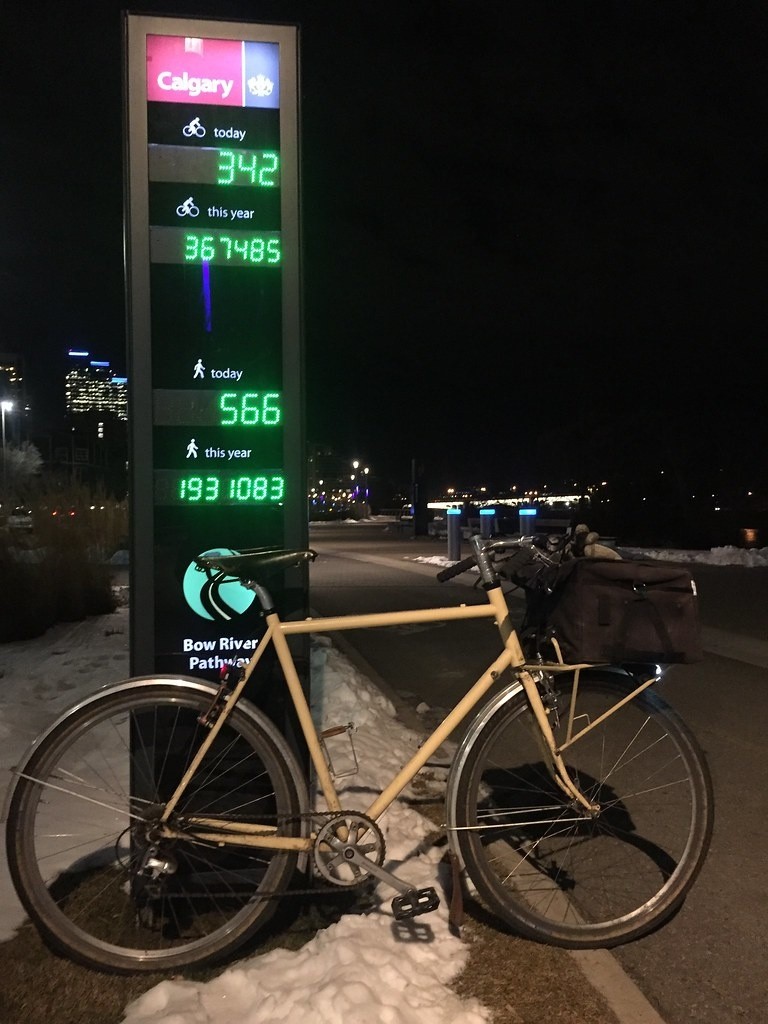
[6,526,714,977]
